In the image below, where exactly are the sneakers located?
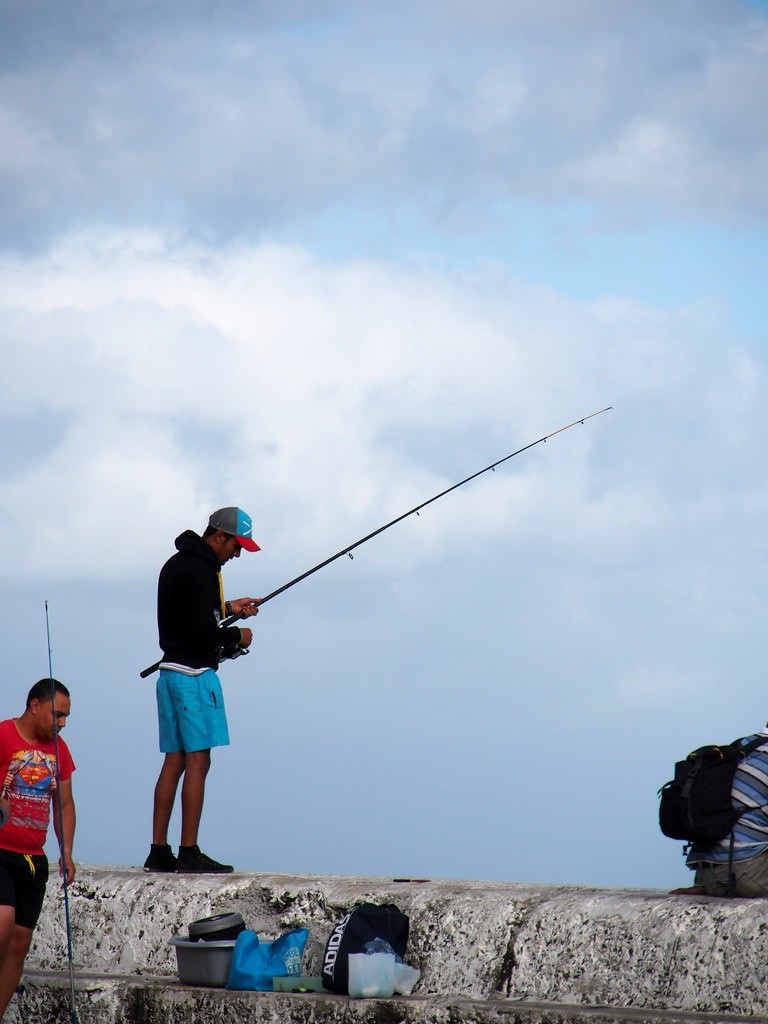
[177,846,234,872]
[143,844,177,873]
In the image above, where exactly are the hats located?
[209,506,261,552]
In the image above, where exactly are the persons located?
[0,678,76,1024]
[669,722,768,898]
[143,507,262,875]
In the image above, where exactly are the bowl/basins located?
[167,934,274,987]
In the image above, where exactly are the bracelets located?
[237,628,243,646]
[225,601,234,616]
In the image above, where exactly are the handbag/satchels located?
[227,928,310,990]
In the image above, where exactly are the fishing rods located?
[45,601,77,1024]
[140,406,614,679]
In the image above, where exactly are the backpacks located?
[657,736,768,856]
[321,903,409,994]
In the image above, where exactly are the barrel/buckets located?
[348,954,396,999]
[188,912,246,942]
